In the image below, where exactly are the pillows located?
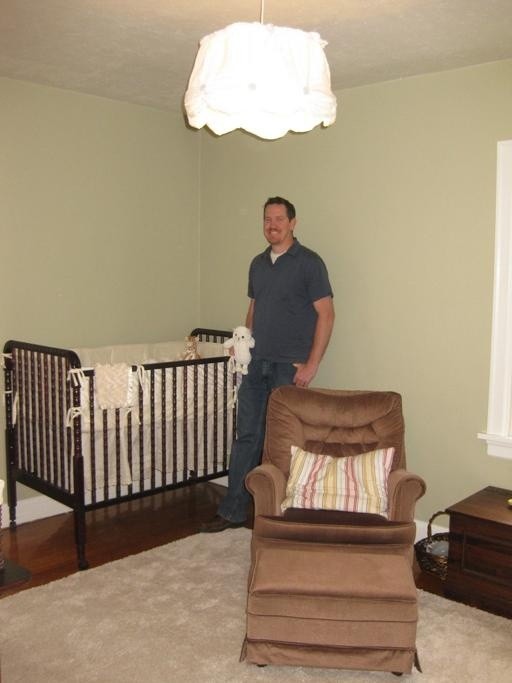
[281,444,396,520]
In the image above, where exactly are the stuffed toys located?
[221,324,256,376]
[182,332,202,360]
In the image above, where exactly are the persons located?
[197,192,336,532]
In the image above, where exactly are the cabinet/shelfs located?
[444,485,512,618]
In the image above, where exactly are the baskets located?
[414,507,451,583]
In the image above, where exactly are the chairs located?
[240,382,426,675]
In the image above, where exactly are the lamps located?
[183,0,338,141]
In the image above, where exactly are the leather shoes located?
[198,512,247,532]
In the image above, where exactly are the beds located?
[2,326,240,572]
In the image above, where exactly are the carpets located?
[0,524,512,682]
